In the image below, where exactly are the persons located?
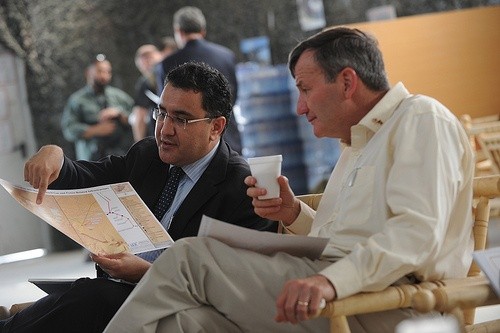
[0,62,278,333]
[61,4,244,159]
[103,29,475,333]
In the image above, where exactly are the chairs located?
[277,174,500,333]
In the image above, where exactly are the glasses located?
[152,107,211,130]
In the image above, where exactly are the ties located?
[150,165,186,222]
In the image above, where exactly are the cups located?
[247,154,283,201]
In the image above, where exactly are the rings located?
[298,301,309,305]
